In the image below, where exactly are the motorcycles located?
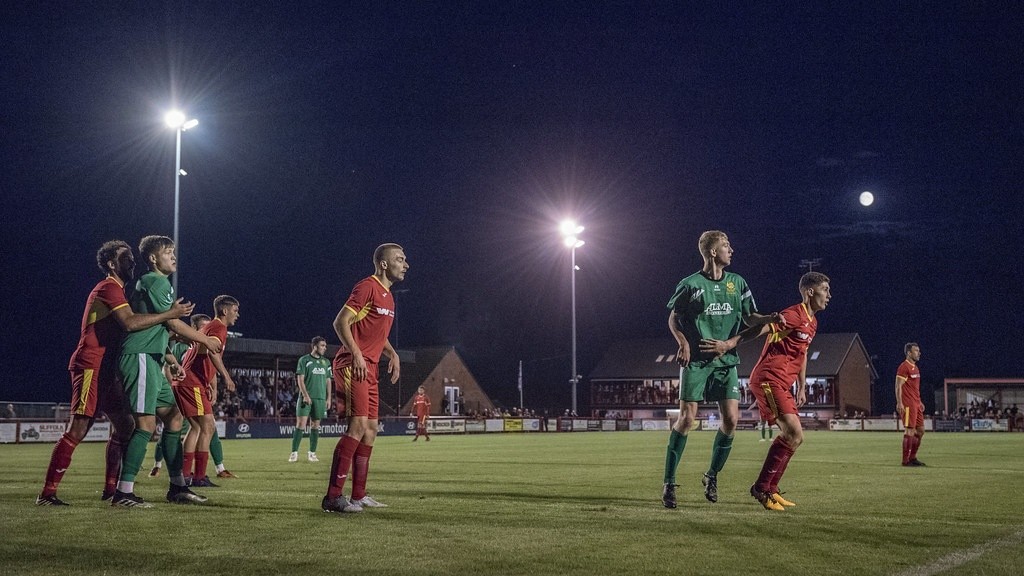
[21,426,40,439]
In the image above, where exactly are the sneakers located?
[111,489,154,508]
[662,482,681,509]
[217,470,239,479]
[102,489,113,501]
[166,482,208,504]
[773,487,796,506]
[701,472,718,503]
[289,452,298,462]
[750,483,785,511]
[902,458,926,466]
[148,466,161,477]
[184,472,221,487]
[308,451,319,462]
[349,495,388,508]
[36,493,70,507]
[322,495,364,514]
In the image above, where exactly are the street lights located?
[163,108,199,303]
[558,218,583,416]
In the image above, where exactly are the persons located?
[410,386,432,441]
[661,231,787,510]
[320,242,410,514]
[564,409,578,417]
[6,403,16,418]
[557,415,563,431]
[592,382,681,419]
[834,410,866,419]
[289,336,332,463]
[895,343,927,466]
[698,272,831,510]
[212,369,341,422]
[464,407,550,434]
[456,390,465,416]
[441,395,449,415]
[959,396,1019,432]
[35,234,241,508]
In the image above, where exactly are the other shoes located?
[412,438,417,441]
[769,438,774,441]
[759,438,766,441]
[425,437,430,441]
